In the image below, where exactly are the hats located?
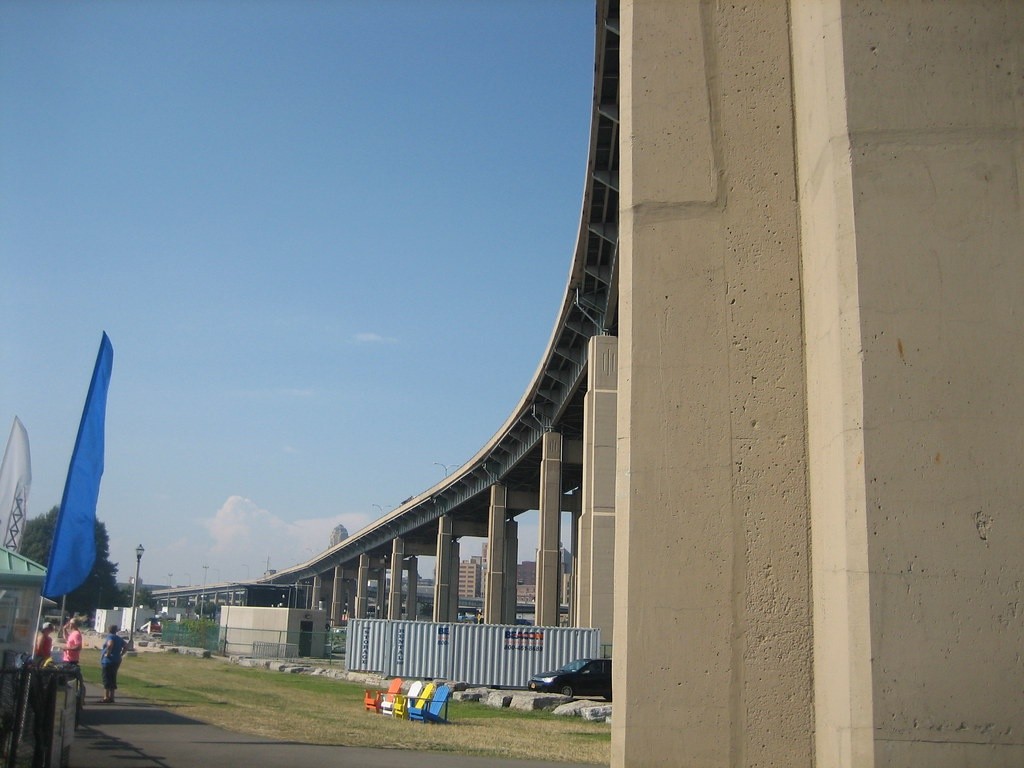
[42,622,54,629]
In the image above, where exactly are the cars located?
[526,656,611,702]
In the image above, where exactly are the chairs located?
[381,681,422,716]
[407,685,450,723]
[394,682,435,719]
[364,678,403,712]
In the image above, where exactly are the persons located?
[61,617,88,696]
[34,621,54,656]
[99,625,130,703]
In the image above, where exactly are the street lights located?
[200,566,210,620]
[373,503,392,517]
[433,461,460,478]
[168,572,173,615]
[124,542,144,656]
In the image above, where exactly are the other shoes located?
[98,698,114,703]
